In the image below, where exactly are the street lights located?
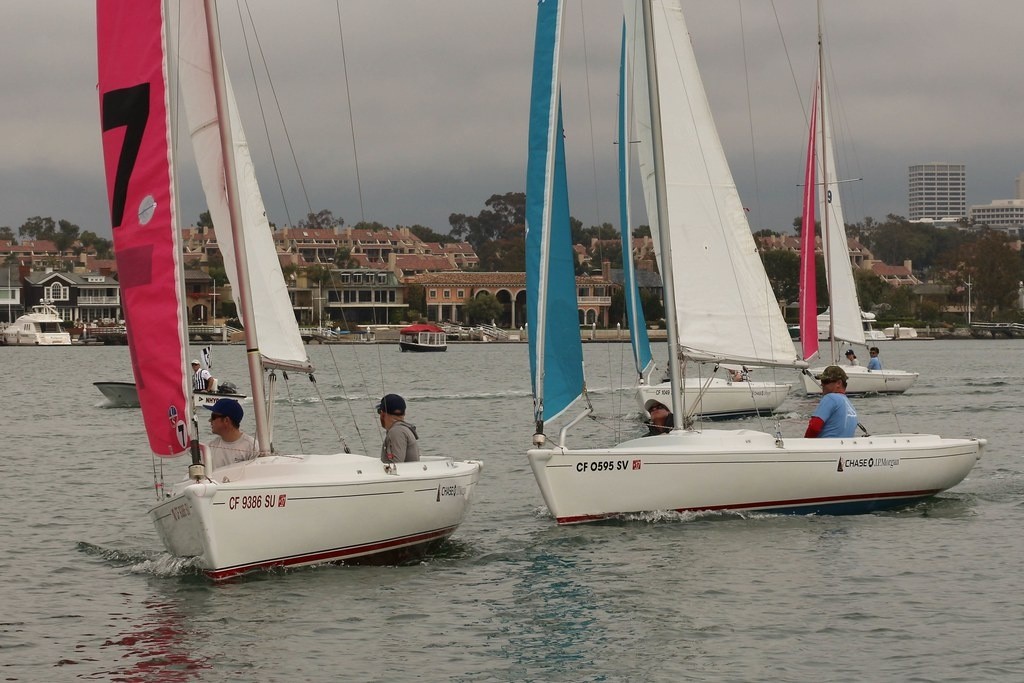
[963,281,973,326]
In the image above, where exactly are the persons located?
[642,399,674,437]
[845,349,860,366]
[867,346,881,370]
[728,369,743,381]
[190,359,214,392]
[202,399,260,471]
[804,366,858,438]
[374,394,420,464]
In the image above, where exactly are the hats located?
[815,366,848,384]
[845,350,853,355]
[377,394,406,415]
[190,359,200,364]
[203,398,243,420]
[645,398,674,415]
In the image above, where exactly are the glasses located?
[870,351,876,355]
[192,363,199,366]
[821,378,837,385]
[378,408,382,414]
[211,413,224,421]
[648,404,669,414]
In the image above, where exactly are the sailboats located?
[616,16,807,426]
[303,247,376,340]
[527,0,989,529]
[95,0,486,582]
[793,3,920,398]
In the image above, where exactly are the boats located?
[787,305,893,341]
[96,381,247,408]
[0,298,72,346]
[398,322,448,353]
